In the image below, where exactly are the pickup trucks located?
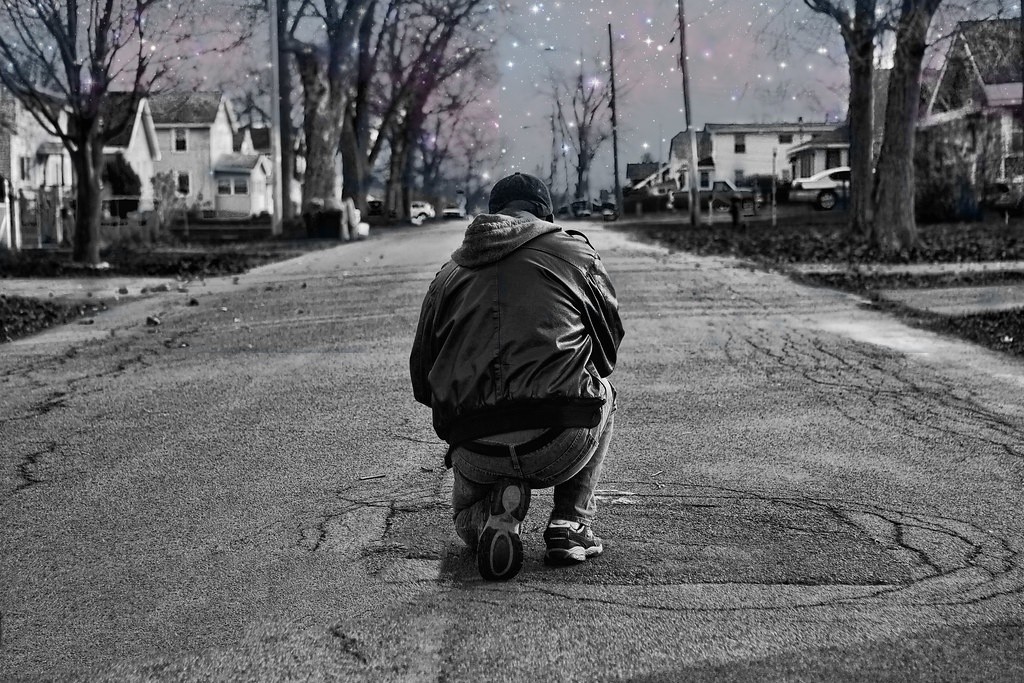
[672,178,762,213]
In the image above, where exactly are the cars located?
[366,194,383,215]
[410,201,436,223]
[558,199,616,218]
[788,166,877,210]
[442,203,468,218]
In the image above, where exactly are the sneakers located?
[543,514,604,565]
[477,476,531,583]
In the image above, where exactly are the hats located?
[488,172,553,217]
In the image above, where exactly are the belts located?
[459,426,565,458]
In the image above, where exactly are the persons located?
[409,172,625,581]
